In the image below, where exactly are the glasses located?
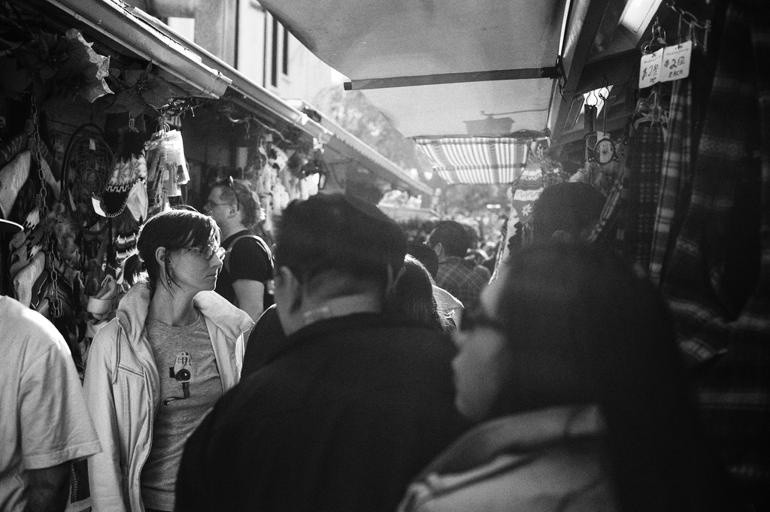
[460,304,504,331]
[208,175,240,210]
[174,244,226,259]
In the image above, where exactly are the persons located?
[84,210,254,511]
[404,218,494,325]
[0,218,103,512]
[176,194,457,512]
[397,239,668,511]
[204,177,290,376]
